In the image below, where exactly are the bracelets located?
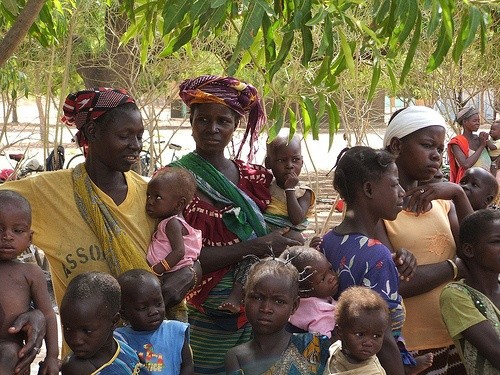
[151,264,164,277]
[444,258,458,280]
[161,259,170,271]
[181,267,196,292]
[284,188,296,193]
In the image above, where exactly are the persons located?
[322,285,391,375]
[458,166,498,210]
[443,105,500,211]
[484,118,500,175]
[0,86,159,375]
[58,265,195,375]
[158,75,306,375]
[318,144,417,375]
[374,105,474,375]
[438,210,500,375]
[226,241,333,375]
[145,171,199,375]
[280,235,341,339]
[219,127,314,312]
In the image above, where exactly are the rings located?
[420,188,424,193]
